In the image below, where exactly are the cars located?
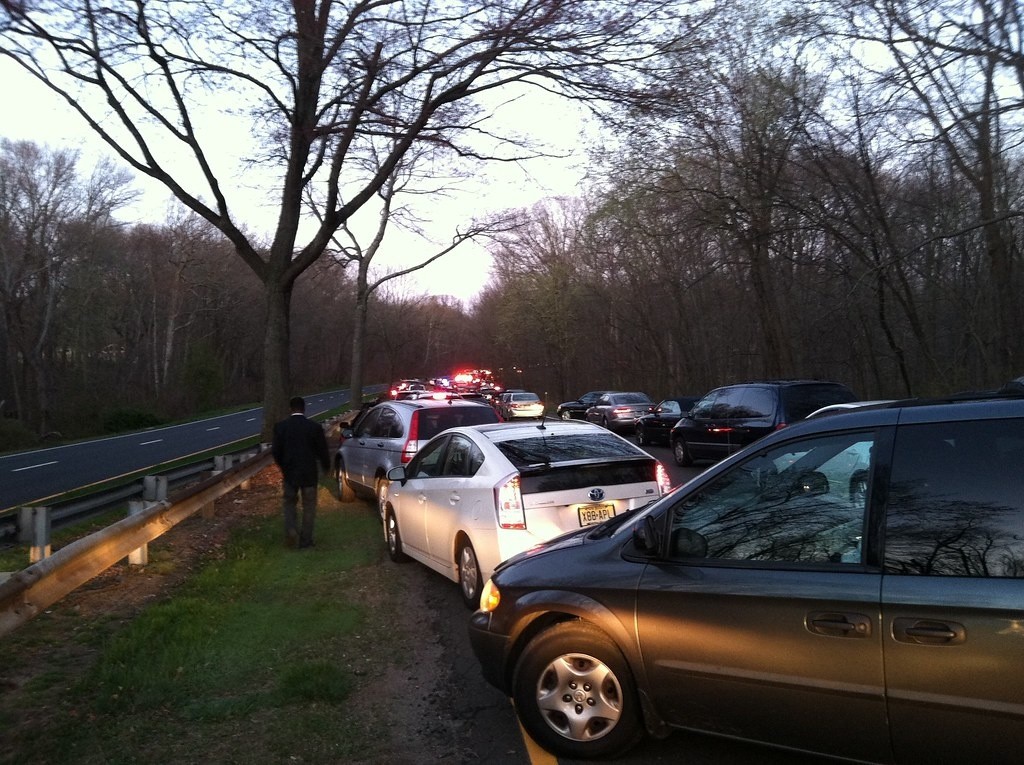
[335,399,506,525]
[583,391,658,432]
[391,369,528,414]
[500,392,545,420]
[634,397,705,447]
[381,415,674,612]
[556,390,619,420]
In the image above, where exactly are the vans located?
[466,379,1023,765]
[669,381,860,467]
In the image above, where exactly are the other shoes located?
[299,540,315,549]
[288,529,297,547]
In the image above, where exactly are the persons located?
[271,397,331,549]
